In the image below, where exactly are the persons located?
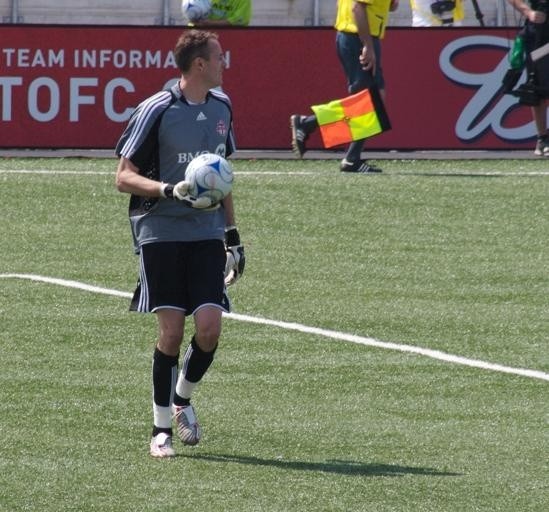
[185,0,251,28]
[286,0,405,175]
[509,1,549,156]
[412,0,468,26]
[113,27,247,460]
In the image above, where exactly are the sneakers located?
[288,113,311,160]
[533,137,549,158]
[147,432,176,458]
[171,399,201,445]
[338,156,383,175]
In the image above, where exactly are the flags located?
[309,82,385,151]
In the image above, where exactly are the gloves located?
[162,181,216,210]
[221,224,246,288]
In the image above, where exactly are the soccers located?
[185,154,233,205]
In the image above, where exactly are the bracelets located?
[223,223,240,246]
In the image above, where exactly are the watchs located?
[164,183,174,200]
